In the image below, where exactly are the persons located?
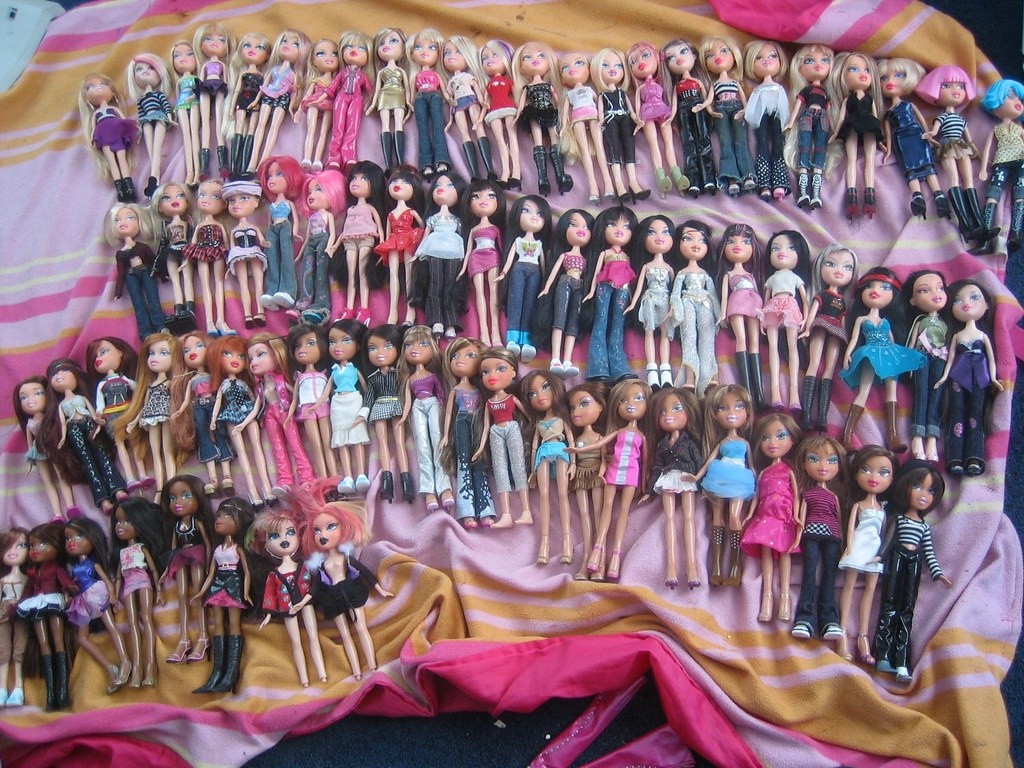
[0,0,1024,768]
